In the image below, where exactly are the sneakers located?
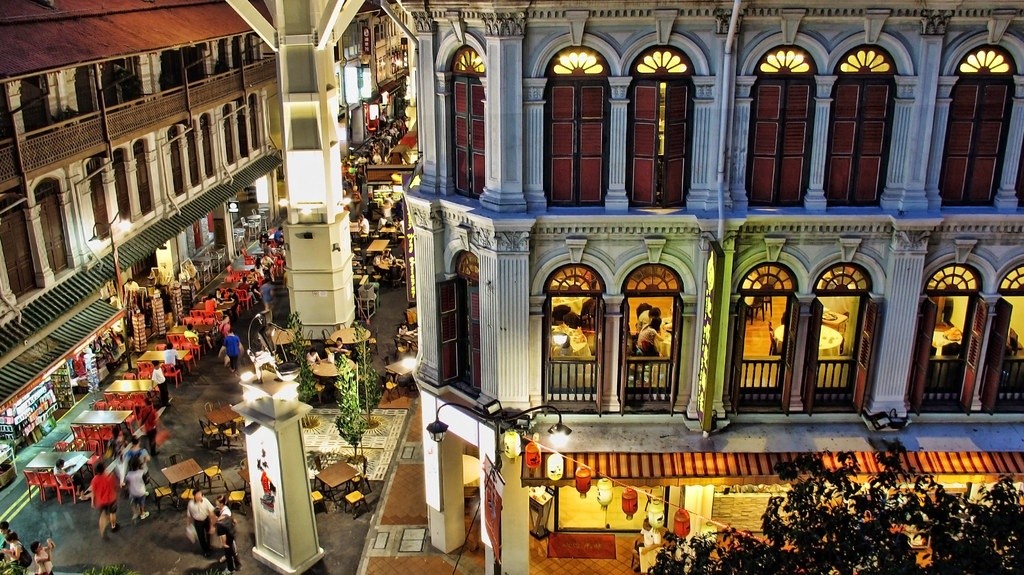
[140,511,150,519]
[131,514,138,520]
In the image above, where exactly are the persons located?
[90,460,121,541]
[124,455,151,521]
[631,518,663,572]
[54,459,89,503]
[542,293,677,379]
[212,508,231,549]
[123,435,151,499]
[31,536,55,575]
[658,530,681,563]
[11,104,422,407]
[137,397,161,458]
[0,520,12,561]
[2,531,26,574]
[211,493,243,574]
[184,488,216,558]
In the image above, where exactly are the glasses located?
[39,543,42,548]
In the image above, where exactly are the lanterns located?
[673,508,692,541]
[523,440,542,469]
[503,430,522,460]
[620,487,639,520]
[572,465,592,498]
[545,453,565,482]
[596,478,613,509]
[647,496,666,534]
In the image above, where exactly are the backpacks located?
[217,516,235,534]
[130,449,143,462]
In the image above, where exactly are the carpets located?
[547,532,616,559]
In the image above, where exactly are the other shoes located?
[235,565,243,570]
[207,544,213,550]
[111,523,121,531]
[220,556,226,563]
[222,568,235,574]
[204,551,208,557]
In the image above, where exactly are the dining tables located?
[550,296,963,365]
[313,459,364,514]
[638,546,666,573]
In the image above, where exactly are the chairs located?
[22,232,289,505]
[344,455,372,494]
[311,453,338,494]
[311,491,329,514]
[294,185,420,402]
[344,475,371,520]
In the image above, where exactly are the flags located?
[482,452,507,565]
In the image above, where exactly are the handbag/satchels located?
[187,524,198,544]
[14,544,32,568]
